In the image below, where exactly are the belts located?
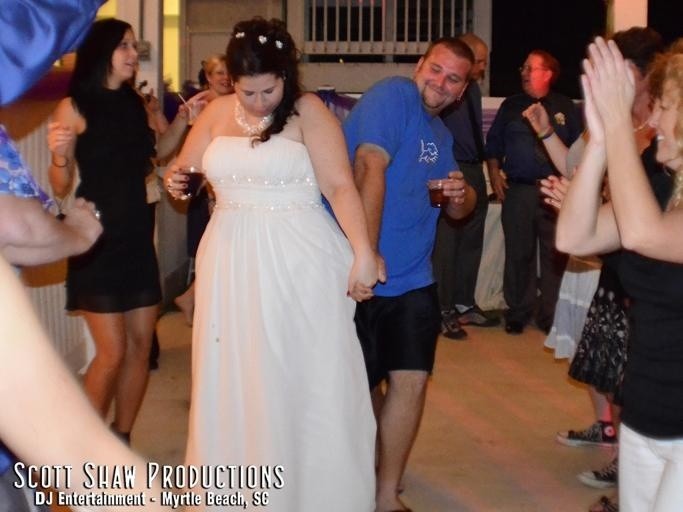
[505,178,546,186]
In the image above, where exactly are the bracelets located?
[50,157,71,171]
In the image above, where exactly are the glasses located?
[519,65,548,72]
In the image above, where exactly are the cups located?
[183,101,207,125]
[176,166,206,198]
[427,179,451,208]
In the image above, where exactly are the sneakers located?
[440,311,467,339]
[456,304,499,327]
[557,421,618,449]
[577,457,618,488]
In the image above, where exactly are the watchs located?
[535,126,556,142]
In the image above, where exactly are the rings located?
[164,180,173,194]
[461,187,467,197]
[93,209,100,219]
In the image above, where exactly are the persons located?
[168,16,383,512]
[54,18,193,451]
[116,47,187,374]
[0,126,171,512]
[491,44,582,337]
[323,38,477,512]
[182,54,241,326]
[436,30,500,342]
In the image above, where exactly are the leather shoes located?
[505,322,524,335]
[539,321,552,336]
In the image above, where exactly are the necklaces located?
[630,114,653,135]
[231,101,273,135]
